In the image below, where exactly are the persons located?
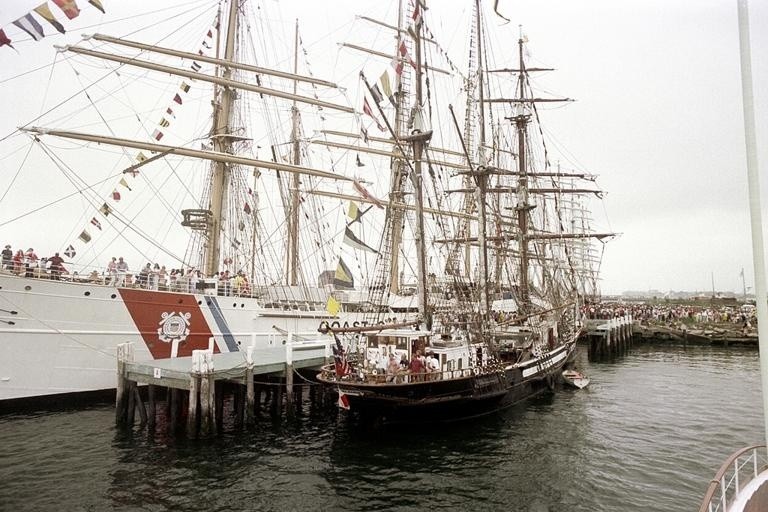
[107,256,250,296]
[447,306,521,328]
[584,302,756,323]
[386,349,441,381]
[0,244,65,280]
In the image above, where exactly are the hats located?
[389,350,434,360]
[5,245,33,252]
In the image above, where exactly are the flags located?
[1,0,103,50]
